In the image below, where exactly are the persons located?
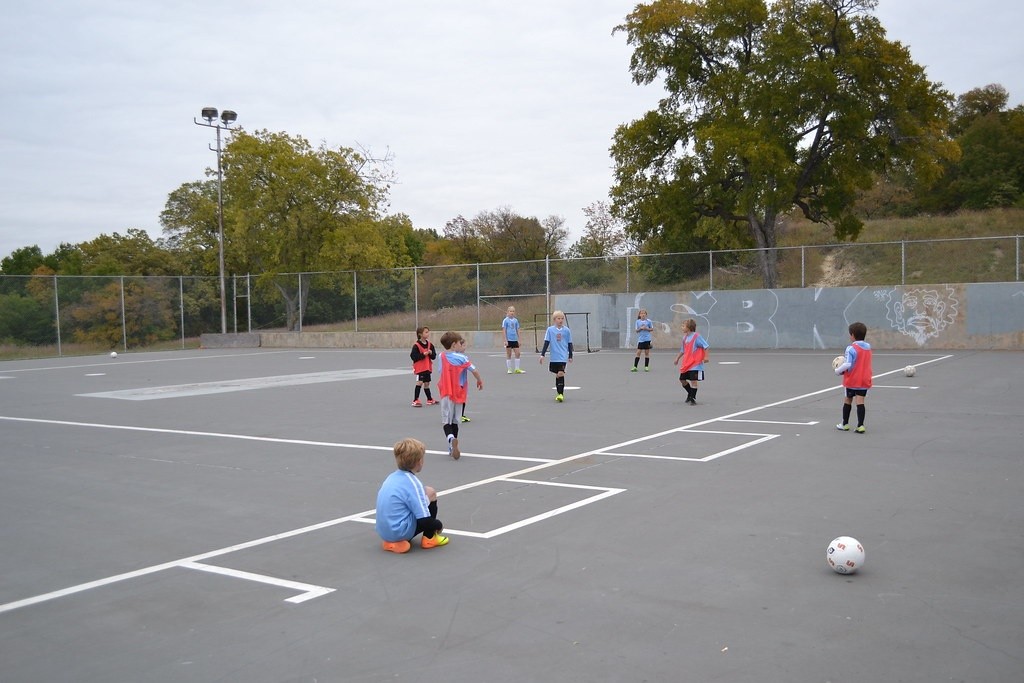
[502,306,526,374]
[376,438,450,553]
[674,319,710,405]
[631,308,654,372]
[410,327,439,406]
[540,310,573,403]
[434,330,484,460]
[835,322,872,432]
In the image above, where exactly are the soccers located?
[826,536,865,575]
[904,365,915,377]
[832,356,845,375]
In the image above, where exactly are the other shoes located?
[631,367,638,372]
[644,367,649,371]
[462,416,471,422]
[507,368,513,374]
[514,369,526,374]
[411,399,422,407]
[690,399,696,405]
[685,394,691,402]
[447,434,460,463]
[426,398,440,405]
[555,394,563,402]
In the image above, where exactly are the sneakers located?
[382,540,410,553]
[421,530,450,548]
[854,425,866,432]
[836,423,850,431]
